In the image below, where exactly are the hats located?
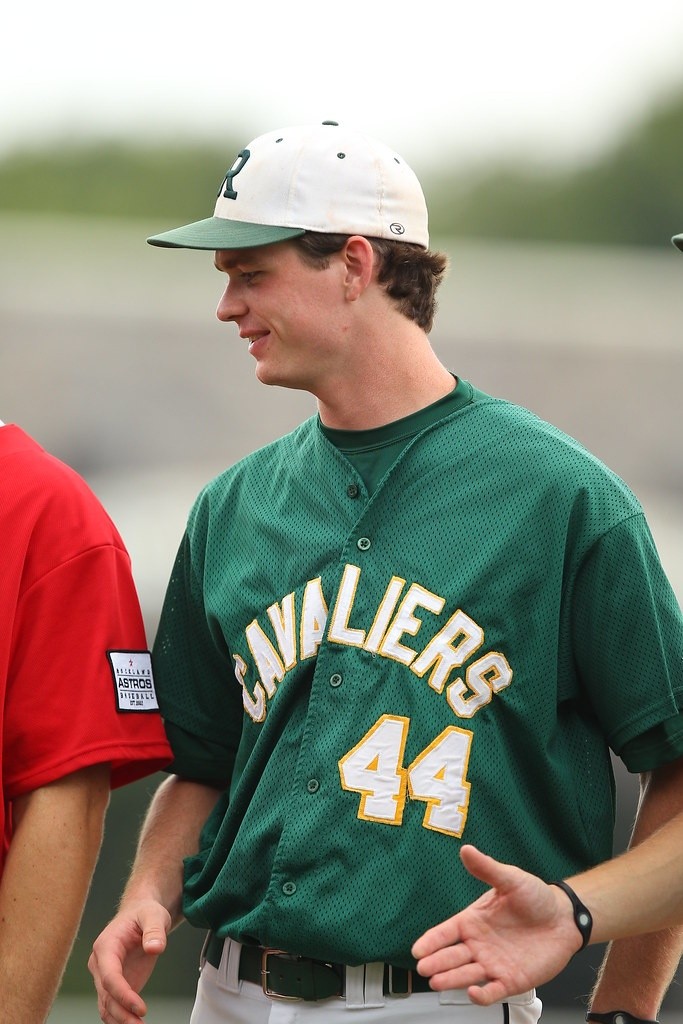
[145,120,428,250]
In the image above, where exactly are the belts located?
[201,936,434,1000]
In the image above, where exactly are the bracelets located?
[546,878,594,953]
[583,1011,661,1024]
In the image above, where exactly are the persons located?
[0,413,176,1022]
[80,113,680,1024]
